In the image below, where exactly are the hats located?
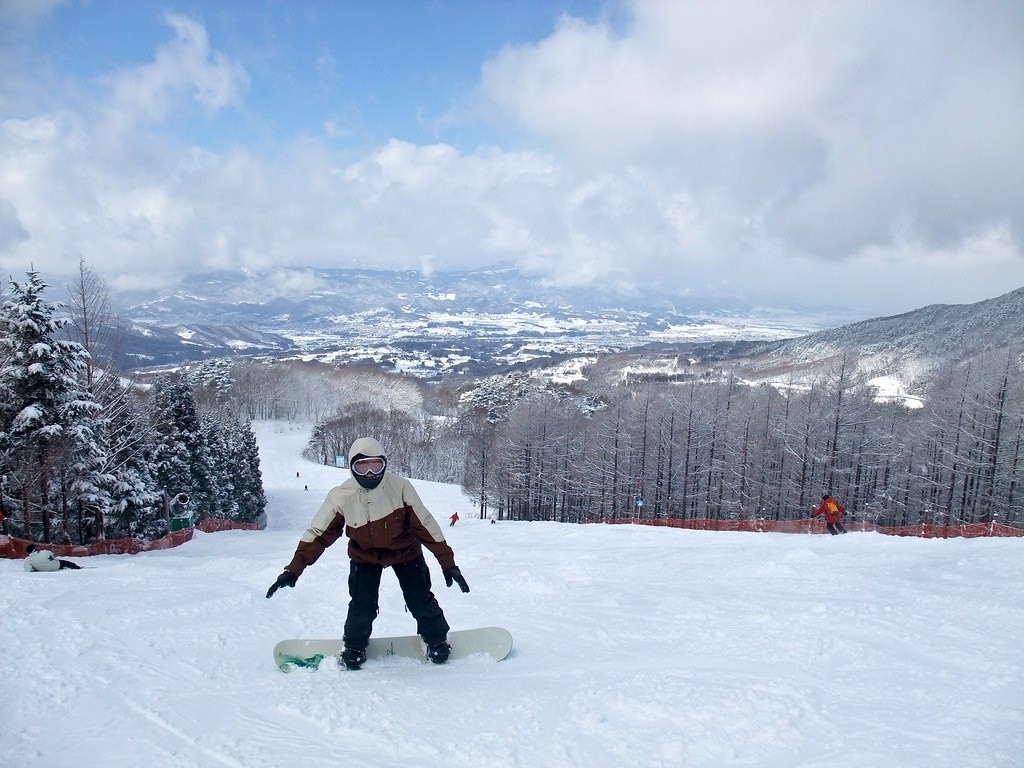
[821,495,829,500]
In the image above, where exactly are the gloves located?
[810,514,814,519]
[442,566,470,593]
[844,513,847,515]
[265,569,299,599]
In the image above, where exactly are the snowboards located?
[273,626,514,674]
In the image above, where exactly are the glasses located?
[351,457,386,475]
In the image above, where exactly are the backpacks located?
[826,501,839,515]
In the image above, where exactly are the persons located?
[490,514,496,525]
[810,494,848,536]
[449,511,460,526]
[266,436,471,670]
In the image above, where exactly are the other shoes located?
[419,635,452,665]
[339,646,368,670]
